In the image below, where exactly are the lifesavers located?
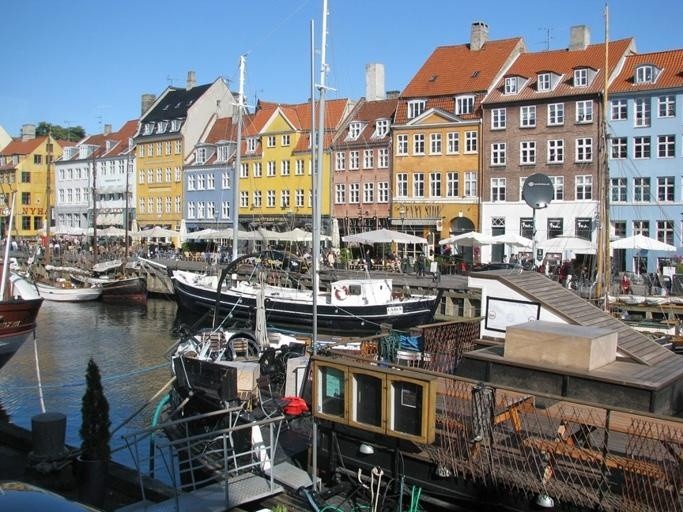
[335,286,349,300]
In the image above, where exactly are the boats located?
[1,359,361,512]
